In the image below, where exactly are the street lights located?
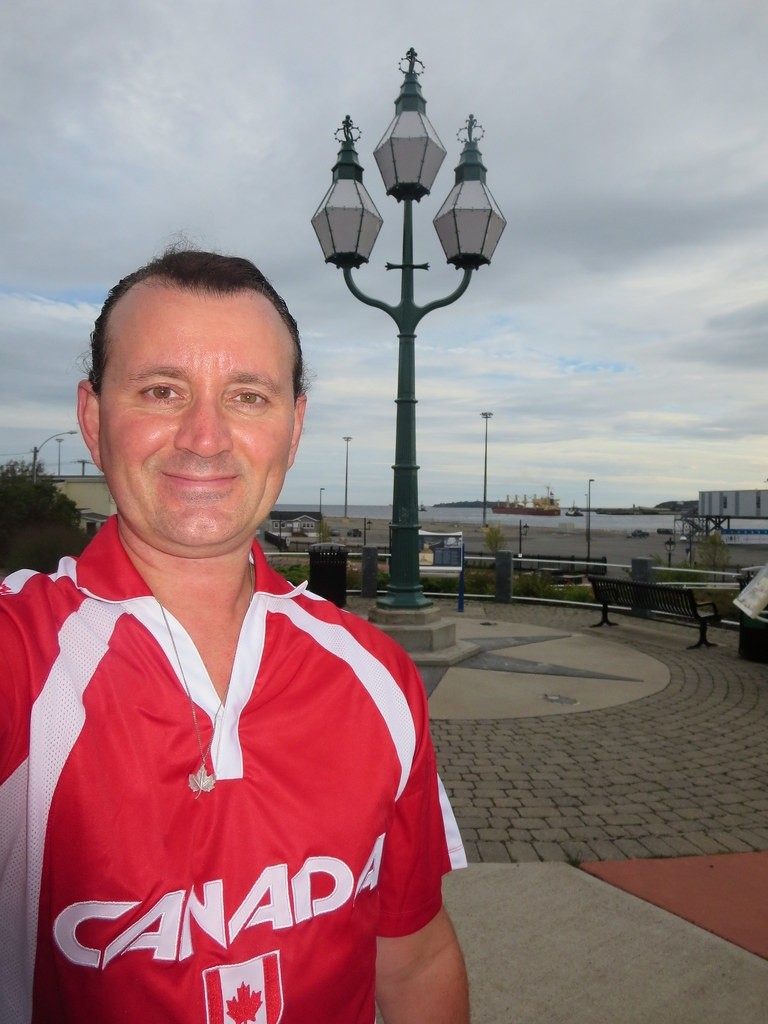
[31,430,78,484]
[319,487,325,521]
[310,45,509,614]
[54,437,66,476]
[665,537,677,568]
[479,411,495,527]
[516,520,531,562]
[363,517,373,545]
[343,436,353,517]
[585,478,595,562]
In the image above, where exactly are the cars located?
[347,527,362,537]
[330,527,340,537]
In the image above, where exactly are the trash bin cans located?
[305,542,350,608]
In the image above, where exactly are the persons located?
[0,251,474,1024]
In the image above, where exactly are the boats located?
[564,501,583,517]
[490,486,562,516]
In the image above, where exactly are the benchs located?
[587,575,721,650]
